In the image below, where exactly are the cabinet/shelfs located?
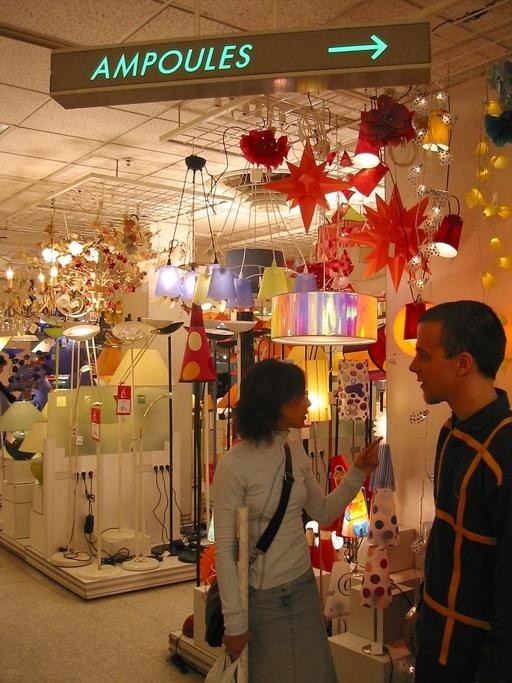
[167,525,432,683]
[1,385,231,601]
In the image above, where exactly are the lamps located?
[0,191,162,343]
[156,72,462,655]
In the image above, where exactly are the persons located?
[401,300,512,682]
[212,357,384,683]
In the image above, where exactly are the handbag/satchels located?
[205,579,225,647]
